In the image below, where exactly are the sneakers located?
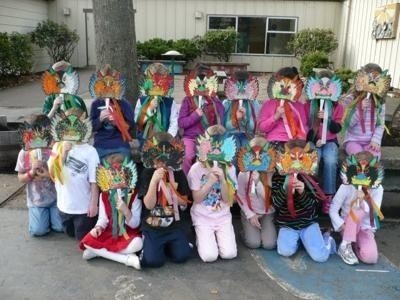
[322,232,337,257]
[82,249,97,260]
[336,241,359,265]
[125,254,141,270]
[322,194,334,216]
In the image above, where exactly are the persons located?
[16,61,392,270]
